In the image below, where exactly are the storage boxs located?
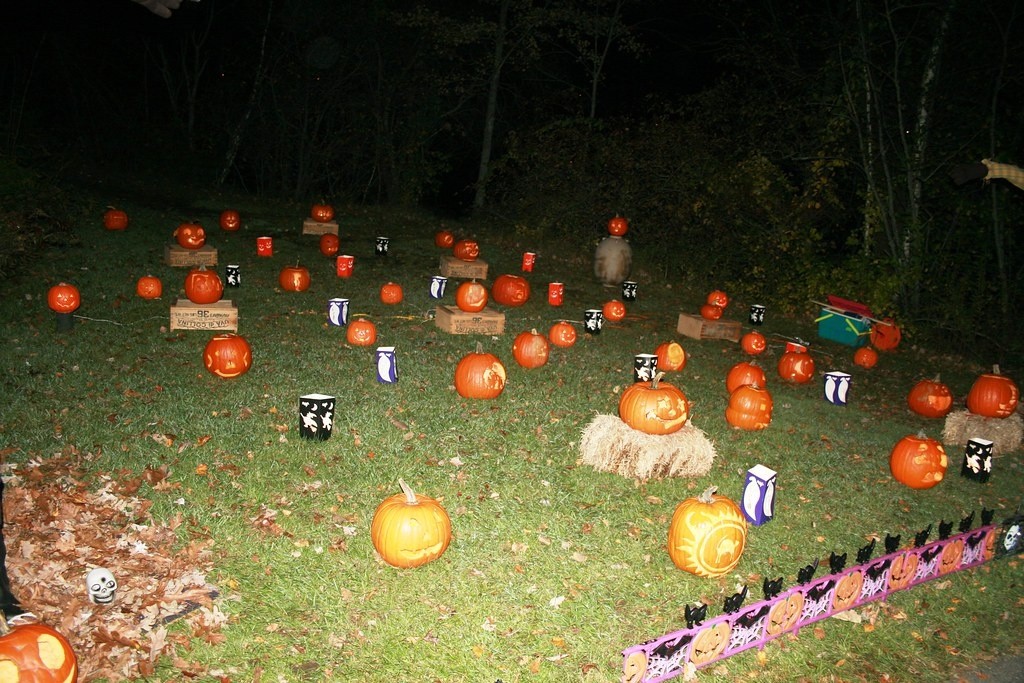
[813,308,872,348]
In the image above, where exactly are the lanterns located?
[618,371,689,436]
[889,427,948,491]
[455,277,489,313]
[607,213,628,236]
[549,320,578,348]
[177,220,206,250]
[706,291,731,309]
[184,262,224,304]
[741,329,767,356]
[491,274,531,307]
[371,477,453,569]
[653,340,687,374]
[512,328,550,369]
[700,299,723,320]
[311,199,334,222]
[203,332,253,379]
[455,341,507,400]
[778,341,815,384]
[48,282,81,314]
[435,229,454,249]
[725,358,767,393]
[453,238,480,262]
[725,380,773,432]
[135,272,163,300]
[908,372,954,417]
[320,229,340,257]
[220,209,241,232]
[278,259,311,292]
[104,205,128,231]
[380,282,403,306]
[854,345,879,369]
[0,610,79,683]
[668,486,748,579]
[346,317,377,347]
[966,364,1019,419]
[603,299,627,322]
